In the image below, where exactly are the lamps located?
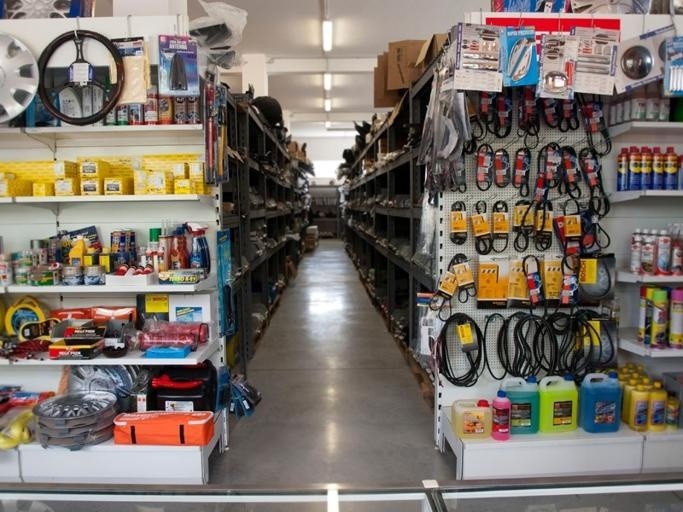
[324,98,333,114]
[320,19,334,54]
[322,70,335,93]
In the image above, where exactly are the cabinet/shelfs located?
[346,93,434,406]
[604,12,683,479]
[0,15,240,483]
[243,97,309,367]
